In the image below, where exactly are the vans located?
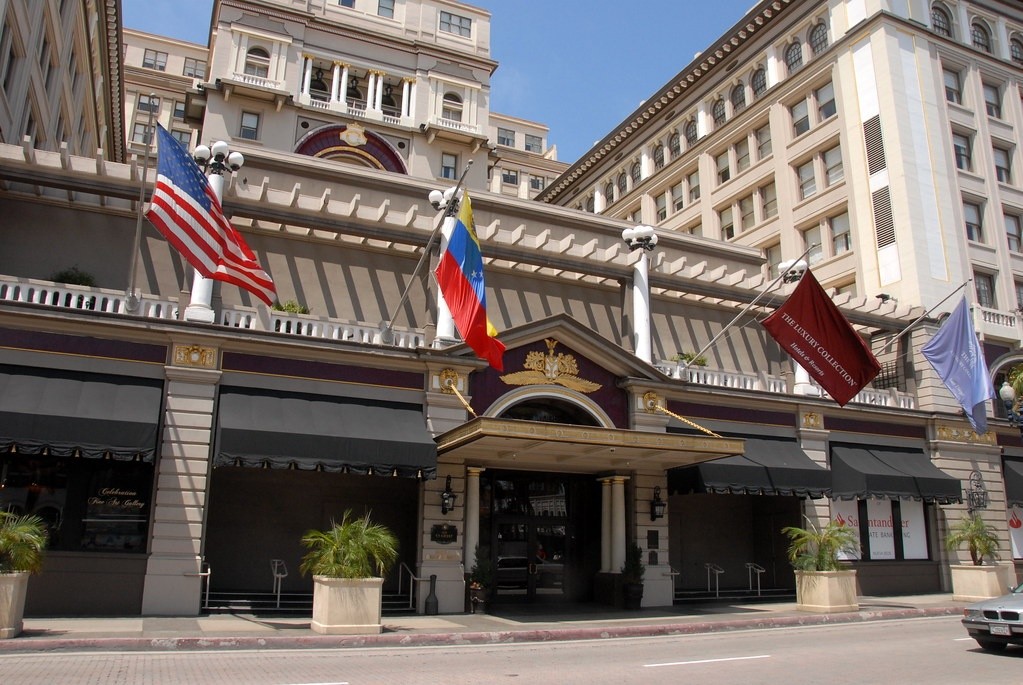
[0,473,69,535]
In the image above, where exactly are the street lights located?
[183,139,246,324]
[620,226,659,368]
[777,257,825,395]
[427,186,472,350]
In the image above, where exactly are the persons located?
[533,543,545,576]
[969,472,985,493]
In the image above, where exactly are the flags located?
[430,187,506,373]
[758,266,883,408]
[920,295,998,438]
[144,122,277,307]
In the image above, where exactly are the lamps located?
[429,186,471,217]
[386,78,393,97]
[649,485,667,521]
[194,141,245,176]
[777,259,807,283]
[315,62,324,82]
[351,70,359,89]
[440,473,458,515]
[965,469,987,514]
[622,226,658,251]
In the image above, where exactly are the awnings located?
[826,431,963,505]
[213,371,438,481]
[0,348,168,465]
[667,418,834,499]
[1000,447,1023,508]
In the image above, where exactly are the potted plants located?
[0,504,51,640]
[300,506,401,635]
[781,515,864,613]
[944,510,1011,601]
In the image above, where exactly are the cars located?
[495,556,566,589]
[962,582,1023,653]
